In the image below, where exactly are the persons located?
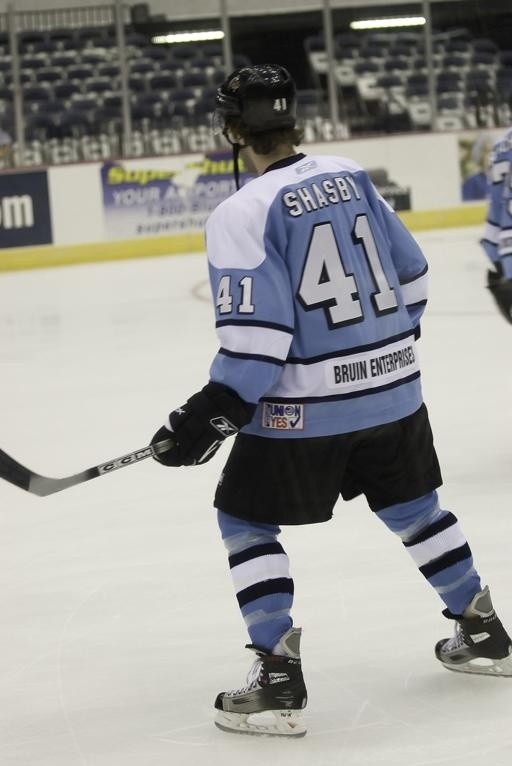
[149,65,512,714]
[479,129,512,325]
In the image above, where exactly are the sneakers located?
[214,627,309,713]
[434,585,512,667]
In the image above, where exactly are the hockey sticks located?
[0,438,179,496]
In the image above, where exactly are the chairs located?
[1,20,512,167]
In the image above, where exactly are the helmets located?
[209,63,300,141]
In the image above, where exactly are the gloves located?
[147,379,259,468]
